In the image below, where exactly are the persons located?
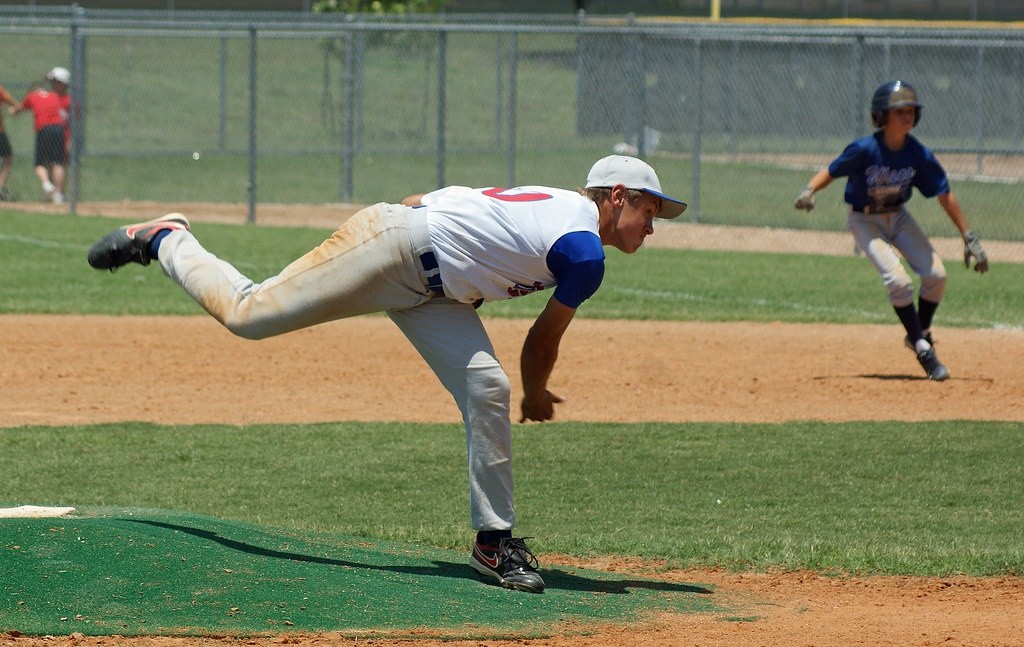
[0,67,72,206]
[794,80,989,381]
[87,155,687,592]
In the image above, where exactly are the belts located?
[853,205,904,212]
[419,251,444,298]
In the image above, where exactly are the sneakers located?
[468,532,546,594]
[87,212,191,273]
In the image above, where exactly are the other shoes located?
[52,192,64,206]
[917,344,951,381]
[41,182,55,203]
[904,335,936,357]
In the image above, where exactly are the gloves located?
[796,189,815,211]
[962,232,989,273]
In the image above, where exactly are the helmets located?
[870,78,923,128]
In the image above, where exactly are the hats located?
[46,67,72,85]
[584,155,688,219]
